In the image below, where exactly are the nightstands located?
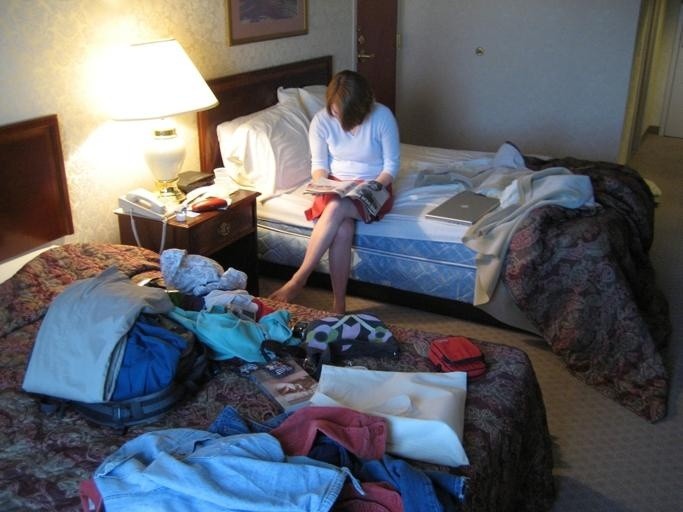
[114,187,263,301]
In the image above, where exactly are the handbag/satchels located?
[308,362,473,470]
[426,335,487,379]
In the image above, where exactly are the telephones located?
[118,189,177,221]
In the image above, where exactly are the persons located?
[266,70,401,314]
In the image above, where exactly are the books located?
[238,355,319,414]
[303,175,390,224]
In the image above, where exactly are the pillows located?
[210,84,369,200]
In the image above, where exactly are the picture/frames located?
[224,0,312,47]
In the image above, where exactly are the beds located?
[1,115,541,511]
[195,53,647,335]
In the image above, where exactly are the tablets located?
[427,188,501,224]
